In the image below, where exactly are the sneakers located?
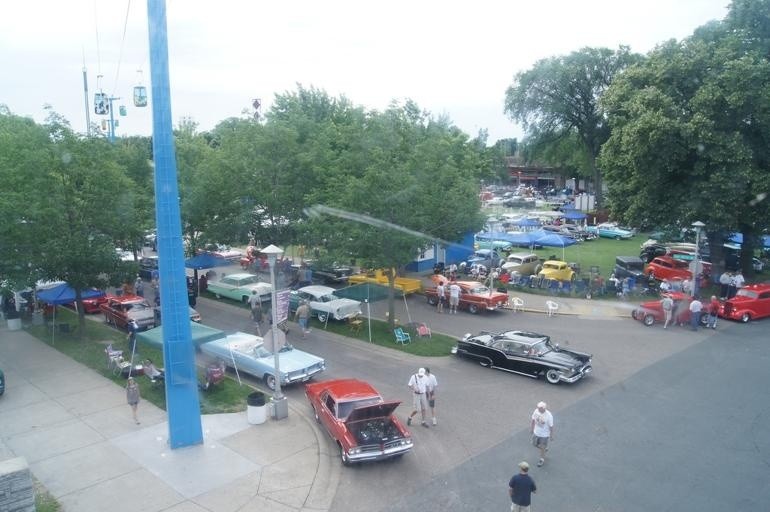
[407,417,436,428]
[538,458,544,467]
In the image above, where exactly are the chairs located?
[104,344,131,378]
[393,327,412,346]
[348,313,363,332]
[416,321,431,339]
[512,297,524,313]
[546,300,558,317]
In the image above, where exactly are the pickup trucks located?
[347,266,423,300]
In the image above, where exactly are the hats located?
[419,368,425,376]
[517,461,529,472]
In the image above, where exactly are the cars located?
[204,273,272,308]
[712,282,770,324]
[632,291,710,327]
[640,220,770,292]
[70,294,113,314]
[100,294,156,332]
[285,284,363,324]
[468,184,646,294]
[112,231,282,278]
[290,258,353,285]
[153,275,197,307]
[304,378,414,466]
[189,305,202,323]
[0,369,5,394]
[426,280,509,316]
[454,329,594,383]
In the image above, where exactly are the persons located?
[249,259,256,274]
[424,367,437,425]
[595,273,629,297]
[249,292,289,333]
[530,402,553,468]
[437,281,460,313]
[662,294,674,329]
[126,377,140,425]
[450,263,458,279]
[508,462,536,512]
[689,296,720,329]
[295,299,312,337]
[470,265,498,286]
[720,270,744,300]
[127,320,136,349]
[188,286,196,308]
[407,366,429,428]
[144,360,165,380]
[200,274,207,291]
[296,265,312,287]
[124,275,160,305]
[641,272,692,295]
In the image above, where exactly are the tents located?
[326,283,412,342]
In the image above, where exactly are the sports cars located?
[200,330,327,391]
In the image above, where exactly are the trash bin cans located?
[246,391,267,425]
[6,311,21,331]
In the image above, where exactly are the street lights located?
[259,245,292,420]
[690,220,706,297]
